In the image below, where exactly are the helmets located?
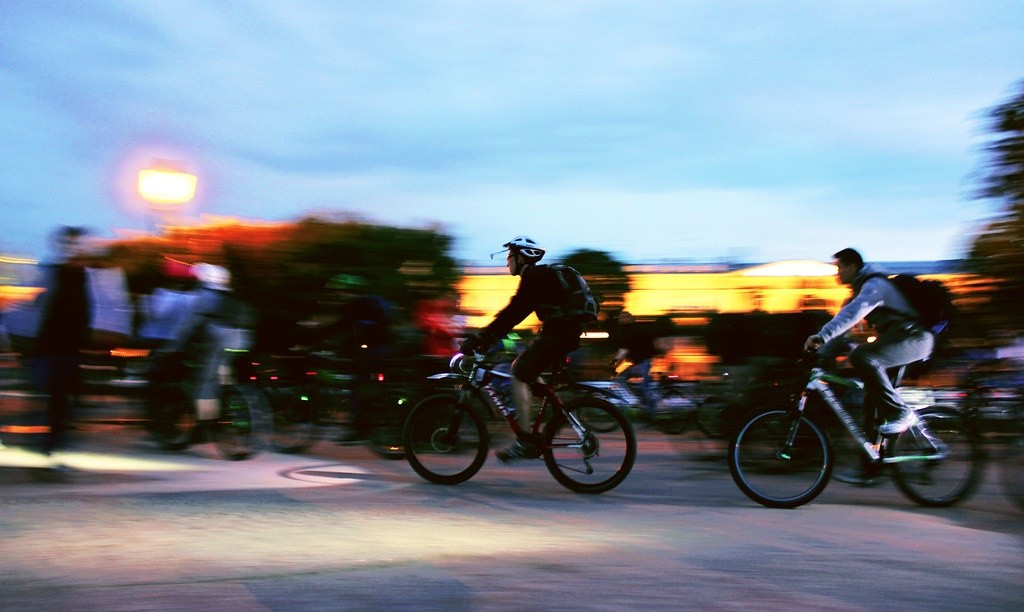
[327,275,370,294]
[195,263,227,289]
[503,237,545,260]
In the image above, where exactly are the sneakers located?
[496,440,537,459]
[881,411,917,435]
[832,469,877,485]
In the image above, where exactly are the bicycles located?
[399,337,640,495]
[722,338,986,508]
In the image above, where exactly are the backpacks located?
[859,271,957,333]
[533,265,600,323]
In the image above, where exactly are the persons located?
[459,236,598,463]
[803,249,938,486]
[609,310,658,423]
[3,220,404,462]
[961,321,1024,412]
